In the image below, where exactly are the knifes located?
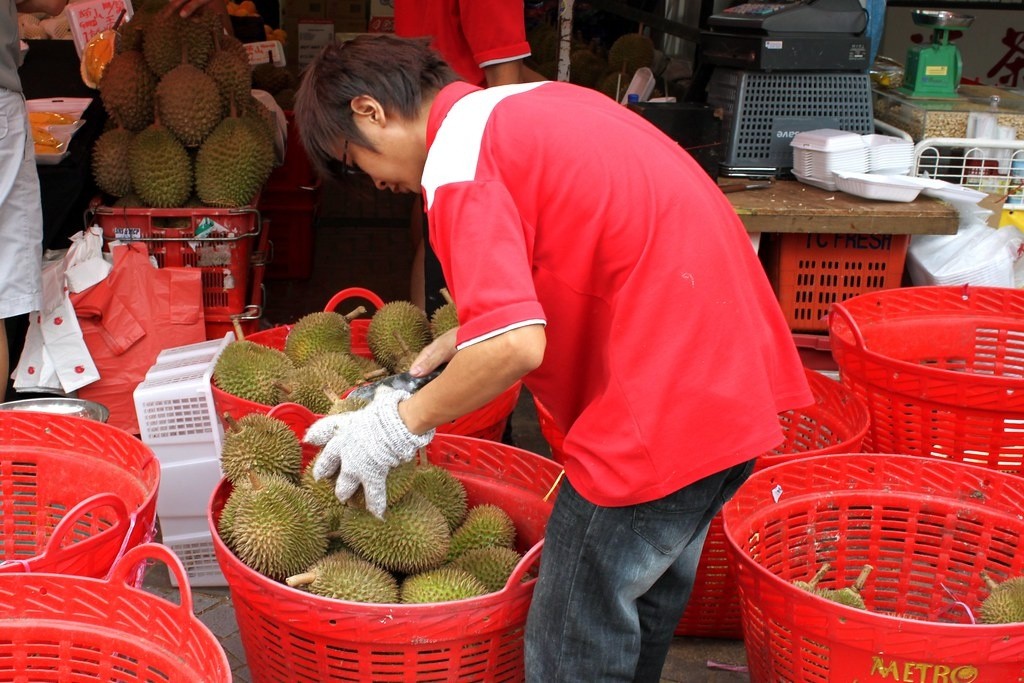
[345,361,449,399]
[718,183,773,193]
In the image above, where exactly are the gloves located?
[302,380,437,518]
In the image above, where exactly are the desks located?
[17,39,119,256]
[715,176,961,235]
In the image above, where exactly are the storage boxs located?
[705,68,875,179]
[285,42,299,65]
[325,0,366,19]
[133,332,235,589]
[832,170,989,204]
[204,219,271,340]
[267,109,322,194]
[334,19,367,33]
[26,97,94,165]
[764,233,910,334]
[89,188,260,316]
[286,19,298,42]
[243,40,286,71]
[787,127,916,192]
[297,18,335,71]
[259,193,320,281]
[285,0,326,18]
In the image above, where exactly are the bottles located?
[627,94,639,103]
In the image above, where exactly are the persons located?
[162,0,275,91]
[0,0,44,403]
[295,34,818,683]
[394,1,530,309]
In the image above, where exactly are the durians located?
[212,287,459,415]
[17,0,281,208]
[777,563,1024,626]
[524,10,663,103]
[218,386,539,604]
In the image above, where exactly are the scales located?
[889,8,976,100]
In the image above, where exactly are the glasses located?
[339,140,363,175]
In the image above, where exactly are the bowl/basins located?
[0,397,109,423]
